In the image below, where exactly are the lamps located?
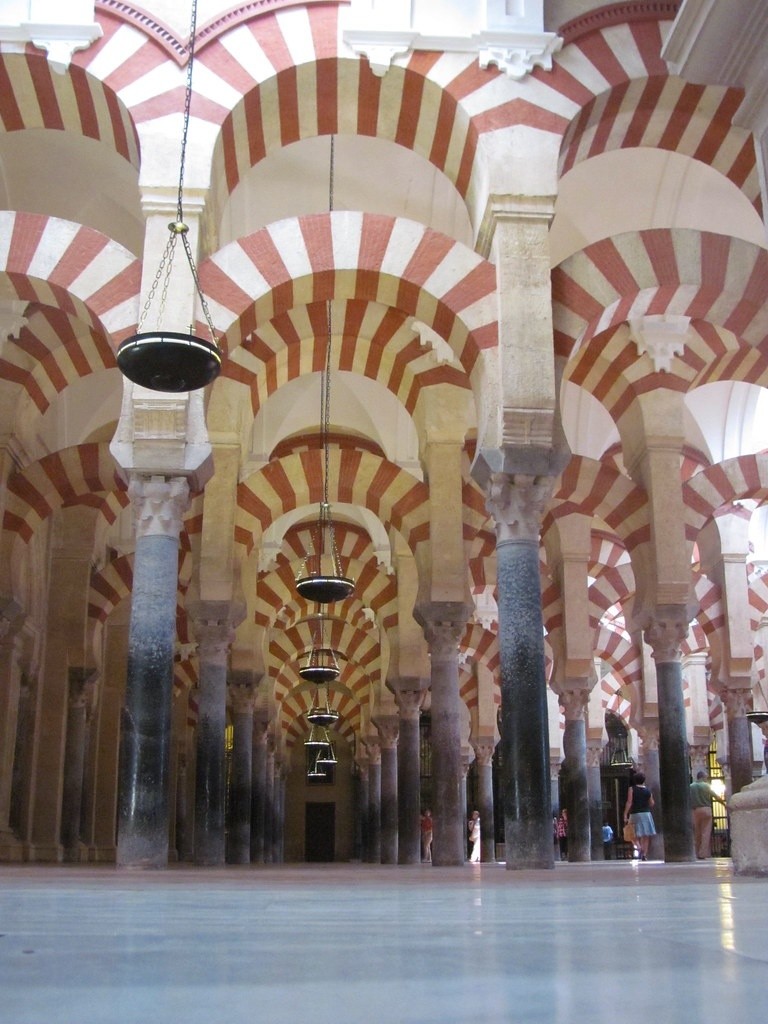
[298,602,340,685]
[117,0,222,394]
[307,682,339,726]
[745,680,768,723]
[292,295,355,604]
[304,723,338,782]
[610,688,633,770]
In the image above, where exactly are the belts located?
[691,804,713,808]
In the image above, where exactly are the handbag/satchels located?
[624,818,636,841]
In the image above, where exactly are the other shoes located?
[638,848,648,860]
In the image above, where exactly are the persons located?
[690,771,728,861]
[623,773,655,860]
[418,807,615,865]
